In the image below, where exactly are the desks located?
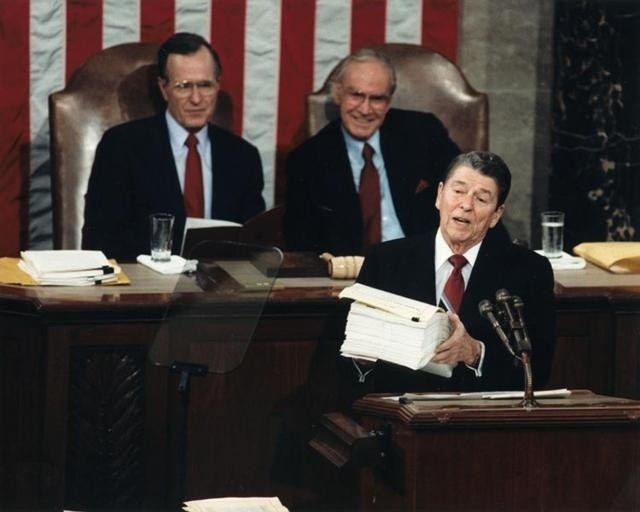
[0,252,640,512]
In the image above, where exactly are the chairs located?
[305,42,490,258]
[48,42,233,259]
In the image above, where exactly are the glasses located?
[170,79,215,94]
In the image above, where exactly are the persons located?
[273,48,514,255]
[75,30,264,262]
[309,150,556,398]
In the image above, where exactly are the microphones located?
[495,288,524,352]
[479,298,515,360]
[512,294,533,352]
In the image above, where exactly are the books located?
[180,198,290,255]
[18,247,123,288]
[178,494,290,512]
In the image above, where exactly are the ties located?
[358,142,381,273]
[183,134,204,219]
[439,254,468,314]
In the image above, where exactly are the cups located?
[149,212,175,262]
[539,210,565,259]
